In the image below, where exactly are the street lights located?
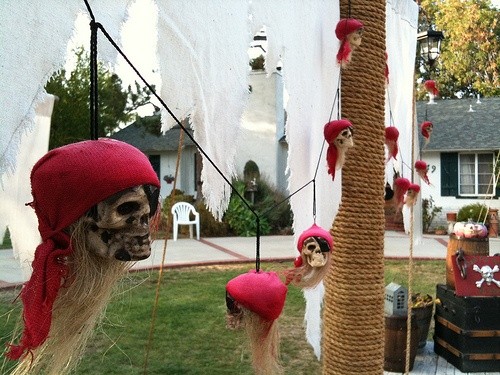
[417,7,444,68]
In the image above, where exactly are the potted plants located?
[436,225,446,235]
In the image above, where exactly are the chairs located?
[171,201,200,241]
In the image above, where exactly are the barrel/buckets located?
[446,235,489,289]
[383,312,418,373]
[408,301,433,350]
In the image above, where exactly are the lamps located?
[418,6,445,67]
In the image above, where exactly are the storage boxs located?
[433,284,500,372]
